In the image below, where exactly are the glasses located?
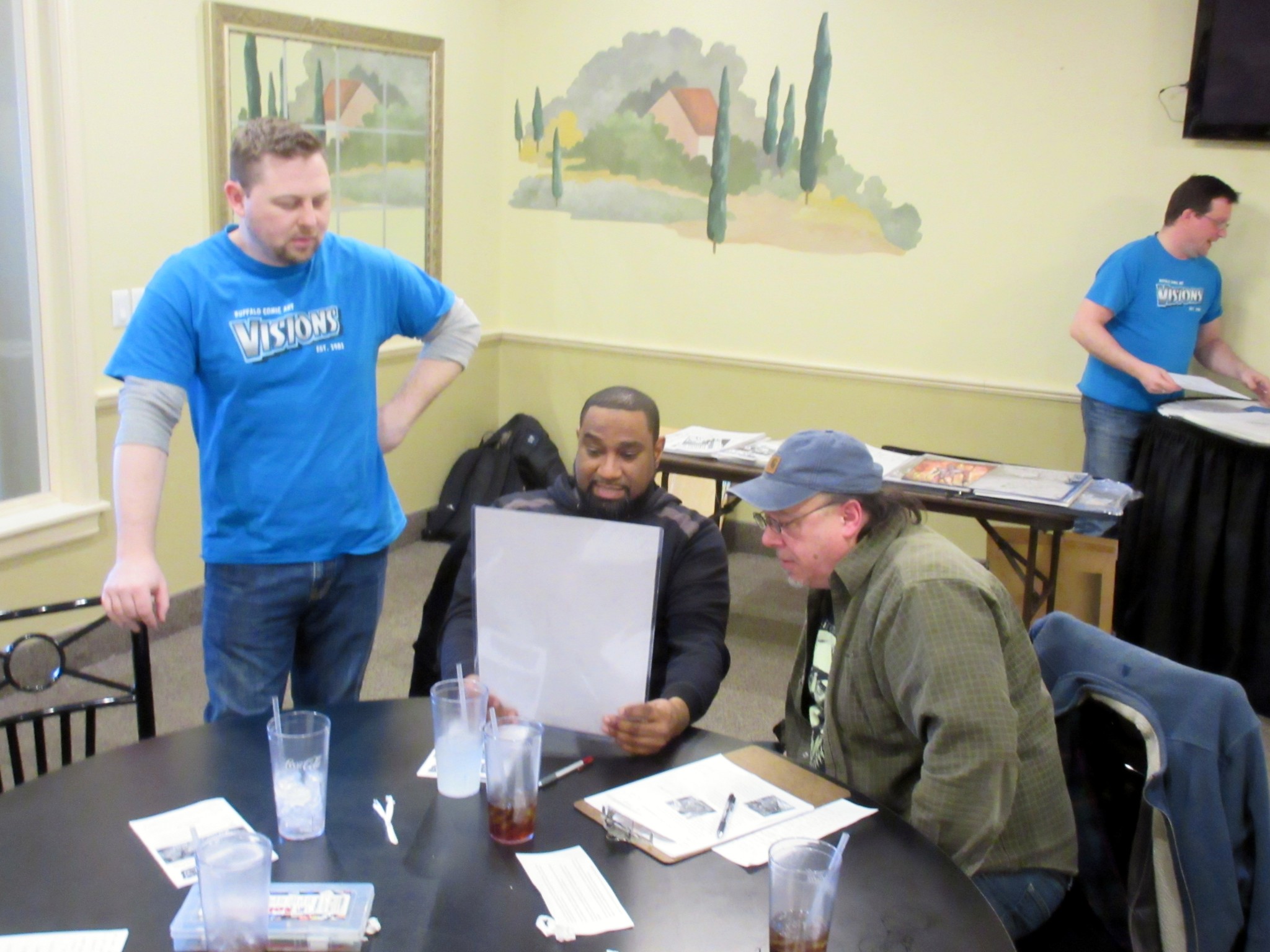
[1196,211,1229,229]
[752,501,837,533]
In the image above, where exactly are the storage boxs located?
[987,529,1118,635]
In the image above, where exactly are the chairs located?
[1013,610,1269,952]
[0,595,157,794]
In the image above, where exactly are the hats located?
[726,430,882,512]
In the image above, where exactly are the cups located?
[483,715,544,845]
[768,836,843,952]
[194,830,273,952]
[430,678,488,798]
[266,710,331,841]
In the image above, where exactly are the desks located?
[2,700,1015,952]
[1111,399,1270,720]
[657,426,1121,631]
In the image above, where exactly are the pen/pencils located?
[716,794,736,838]
[538,755,594,788]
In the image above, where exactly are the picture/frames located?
[203,2,442,282]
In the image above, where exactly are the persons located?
[440,385,729,757]
[101,114,483,724]
[1069,174,1270,539]
[726,429,1080,937]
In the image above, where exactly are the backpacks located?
[421,430,526,539]
